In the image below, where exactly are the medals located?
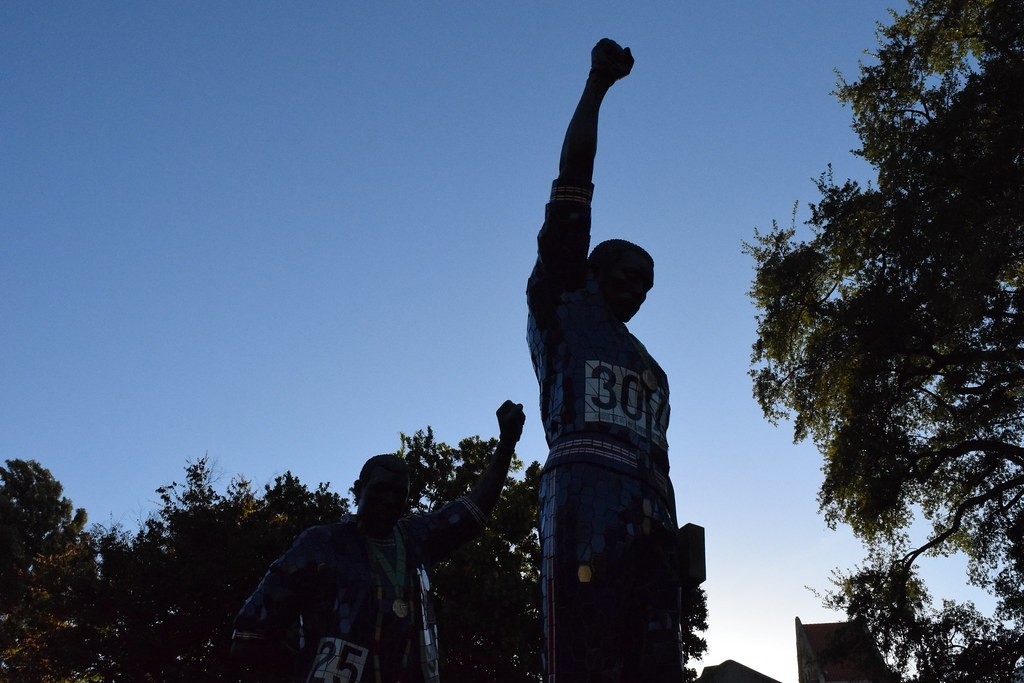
[393,598,408,618]
[642,370,658,391]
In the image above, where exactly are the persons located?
[522,38,684,683]
[229,399,527,683]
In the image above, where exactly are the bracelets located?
[589,61,617,87]
